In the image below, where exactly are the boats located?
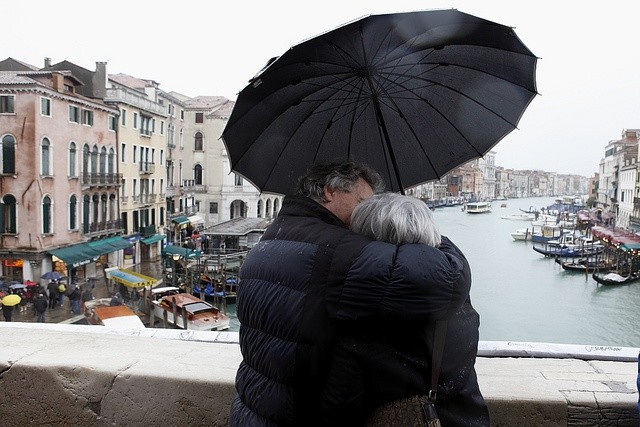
[466,202,491,213]
[511,195,639,285]
[150,292,229,330]
[83,298,144,326]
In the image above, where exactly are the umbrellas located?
[218,7,544,196]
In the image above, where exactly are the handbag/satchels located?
[369,395,441,427]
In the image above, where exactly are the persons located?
[348,190,493,425]
[0,271,93,322]
[227,157,472,427]
[109,281,144,310]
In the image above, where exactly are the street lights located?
[173,254,181,281]
